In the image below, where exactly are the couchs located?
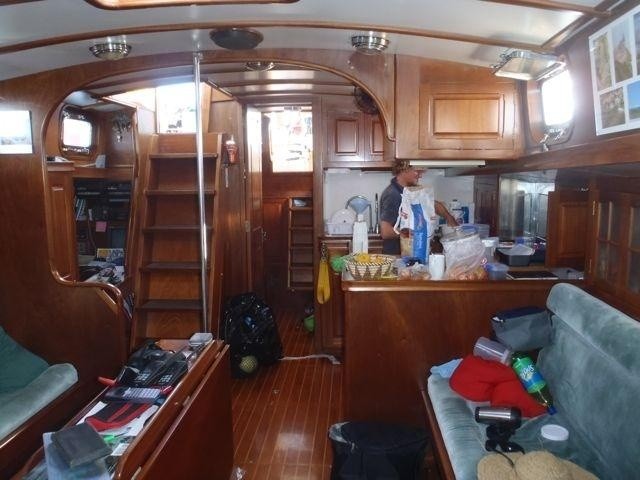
[421,283,639,480]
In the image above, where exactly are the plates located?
[330,208,357,225]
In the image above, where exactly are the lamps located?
[351,36,389,56]
[244,62,275,71]
[91,43,131,61]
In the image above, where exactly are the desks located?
[10,339,233,480]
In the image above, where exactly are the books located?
[50,420,113,470]
[74,179,134,320]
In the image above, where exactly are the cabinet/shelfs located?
[395,55,526,161]
[321,94,395,169]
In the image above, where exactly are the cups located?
[475,406,522,428]
[428,254,446,278]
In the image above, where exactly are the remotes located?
[104,386,161,400]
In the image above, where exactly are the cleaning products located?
[353,214,369,253]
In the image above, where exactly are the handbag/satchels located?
[491,306,554,351]
[328,422,430,479]
[221,293,283,377]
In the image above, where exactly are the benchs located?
[1,325,88,480]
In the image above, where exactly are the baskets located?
[343,254,396,280]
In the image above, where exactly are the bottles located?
[472,336,510,365]
[510,350,557,415]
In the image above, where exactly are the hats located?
[477,450,600,480]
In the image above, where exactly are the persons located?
[378,159,459,258]
[611,35,633,83]
